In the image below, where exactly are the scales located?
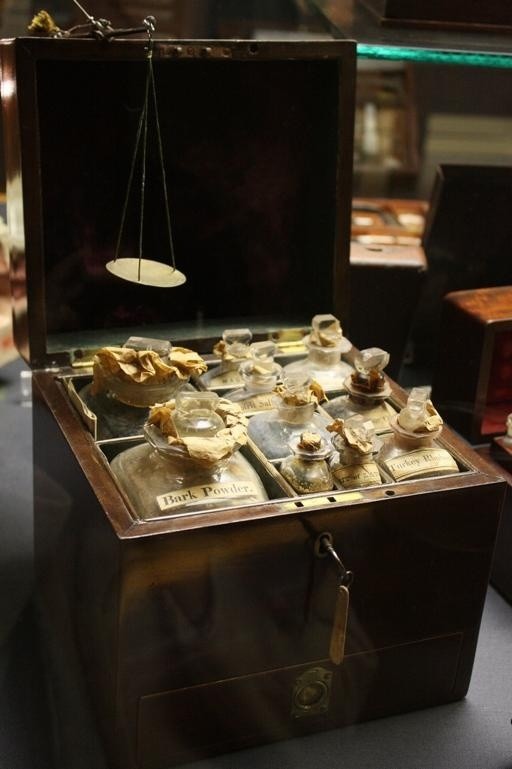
[54,0,187,288]
